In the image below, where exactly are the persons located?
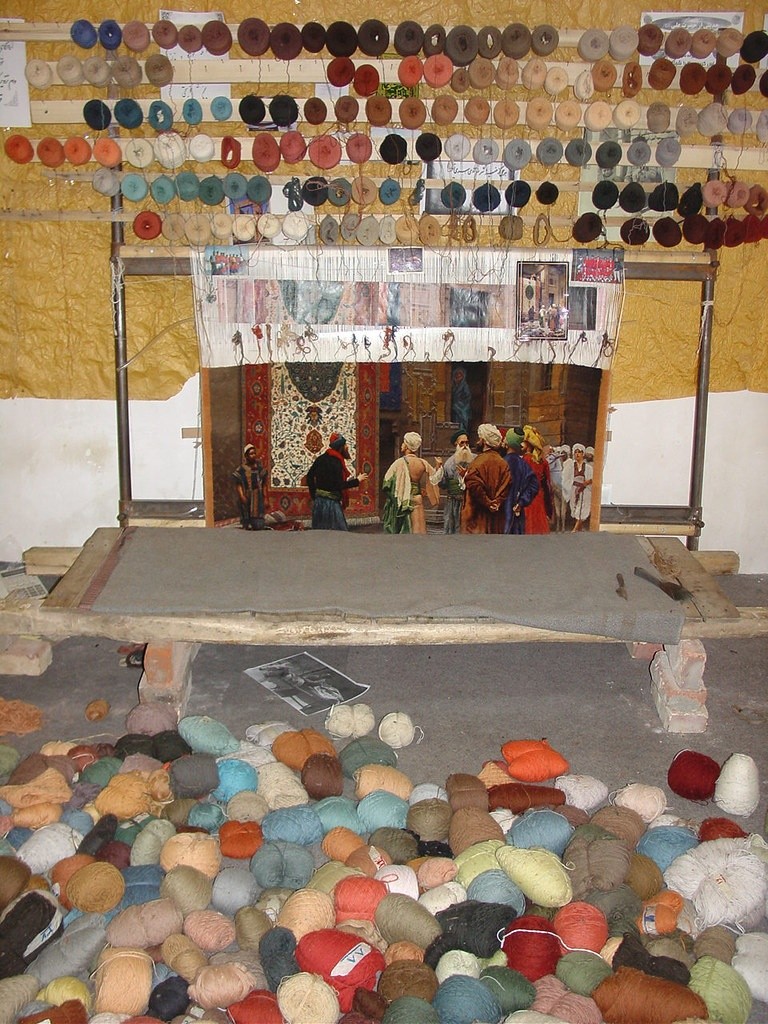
[528,303,564,333]
[231,443,268,531]
[438,423,594,535]
[390,249,421,271]
[381,432,442,534]
[575,252,624,284]
[210,250,244,275]
[307,434,368,531]
[452,366,471,429]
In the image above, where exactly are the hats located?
[244,444,255,454]
[329,433,346,450]
[450,430,466,446]
[478,423,596,456]
[404,432,422,452]
